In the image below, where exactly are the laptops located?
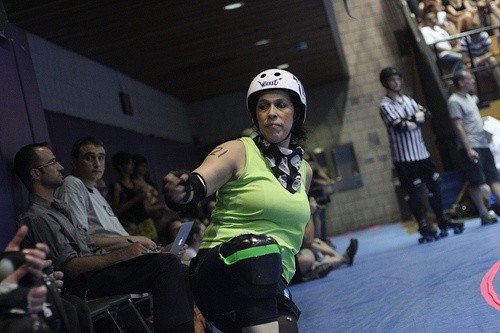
[150,221,194,253]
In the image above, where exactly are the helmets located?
[379,65,402,86]
[246,68,308,125]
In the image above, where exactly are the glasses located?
[37,159,57,170]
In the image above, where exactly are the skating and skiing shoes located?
[438,218,464,236]
[417,224,438,244]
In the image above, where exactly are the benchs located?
[441,35,500,121]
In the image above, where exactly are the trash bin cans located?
[391,167,414,222]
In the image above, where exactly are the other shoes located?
[301,263,333,282]
[345,238,358,265]
[480,215,497,225]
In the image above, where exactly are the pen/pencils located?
[127,238,135,243]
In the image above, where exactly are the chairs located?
[86,291,156,333]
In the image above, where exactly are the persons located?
[0,225,92,333]
[408,0,500,99]
[110,145,217,267]
[379,68,463,236]
[14,141,195,333]
[162,68,358,333]
[448,69,500,225]
[54,134,156,248]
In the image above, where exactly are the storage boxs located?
[393,172,461,220]
